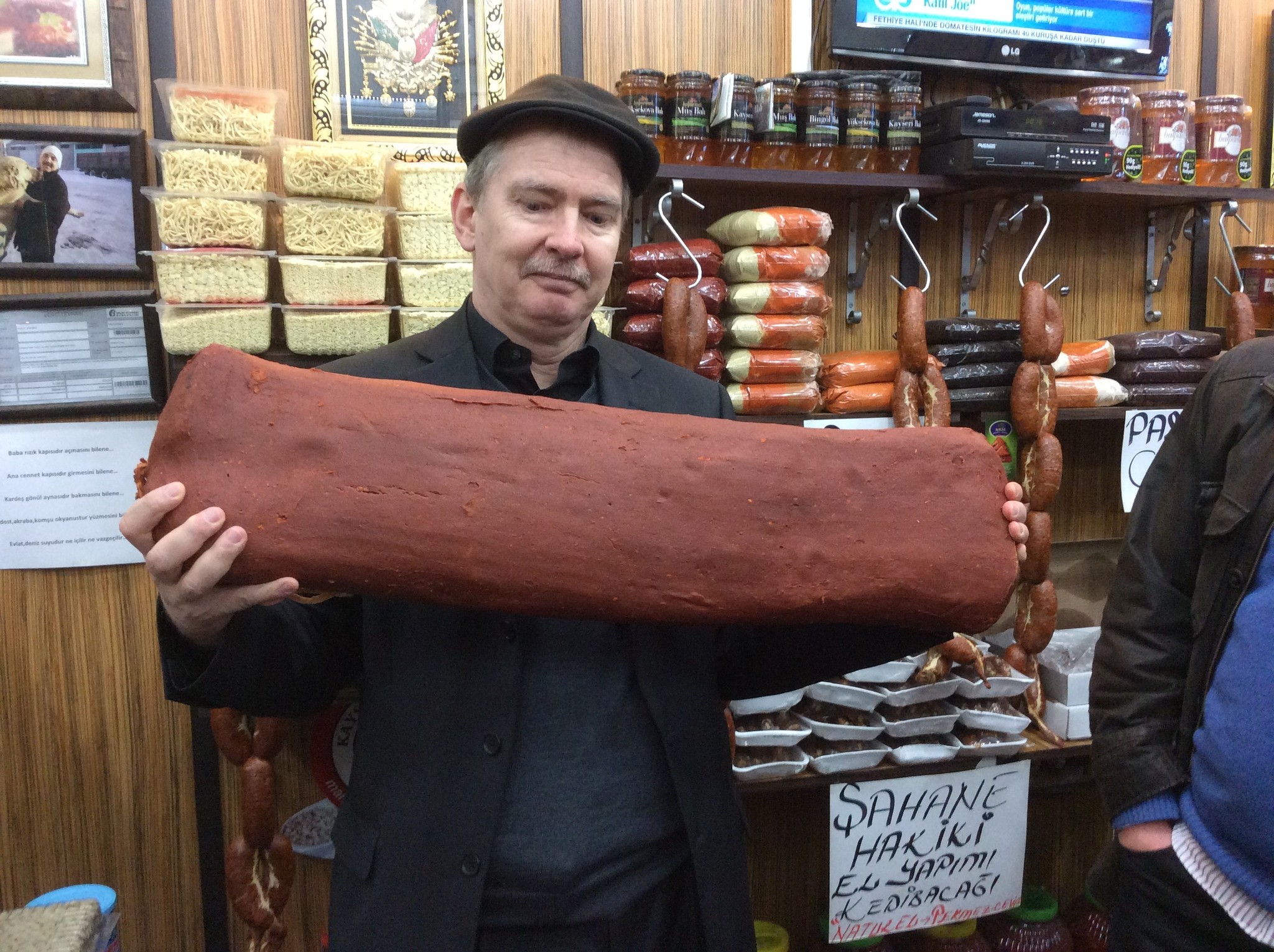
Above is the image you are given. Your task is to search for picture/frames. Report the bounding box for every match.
[0,290,168,418]
[304,0,506,163]
[0,122,154,280]
[0,0,140,113]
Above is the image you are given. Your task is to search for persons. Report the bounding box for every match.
[14,144,72,262]
[1087,332,1274,952]
[117,74,1031,952]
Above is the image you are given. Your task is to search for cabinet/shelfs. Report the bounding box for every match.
[658,165,952,430]
[962,179,1272,423]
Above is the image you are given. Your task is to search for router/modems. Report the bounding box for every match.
[914,138,1115,177]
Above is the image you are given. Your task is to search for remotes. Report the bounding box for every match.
[913,95,994,111]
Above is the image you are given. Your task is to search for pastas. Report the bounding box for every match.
[136,74,479,363]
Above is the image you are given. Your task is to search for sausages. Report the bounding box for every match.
[1228,288,1259,351]
[663,279,711,372]
[889,279,1064,745]
[205,704,299,950]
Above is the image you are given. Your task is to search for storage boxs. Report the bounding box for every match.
[985,625,1101,740]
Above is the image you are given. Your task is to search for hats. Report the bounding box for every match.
[456,74,662,198]
[39,145,64,169]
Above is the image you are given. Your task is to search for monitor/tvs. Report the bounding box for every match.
[830,0,1172,82]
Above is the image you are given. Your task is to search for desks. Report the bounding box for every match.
[742,722,1112,952]
